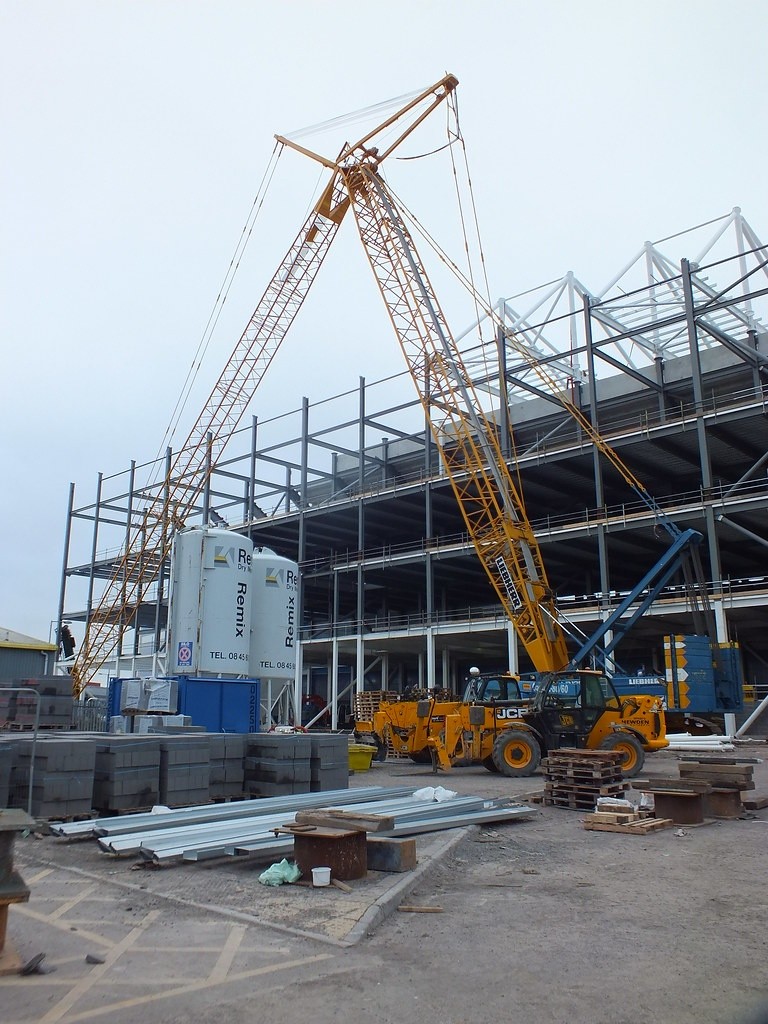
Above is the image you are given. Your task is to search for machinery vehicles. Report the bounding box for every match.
[64,71,748,786]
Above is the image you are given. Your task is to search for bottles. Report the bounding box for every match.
[280,728,302,734]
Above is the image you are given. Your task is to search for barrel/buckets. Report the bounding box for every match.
[311,867,331,886]
